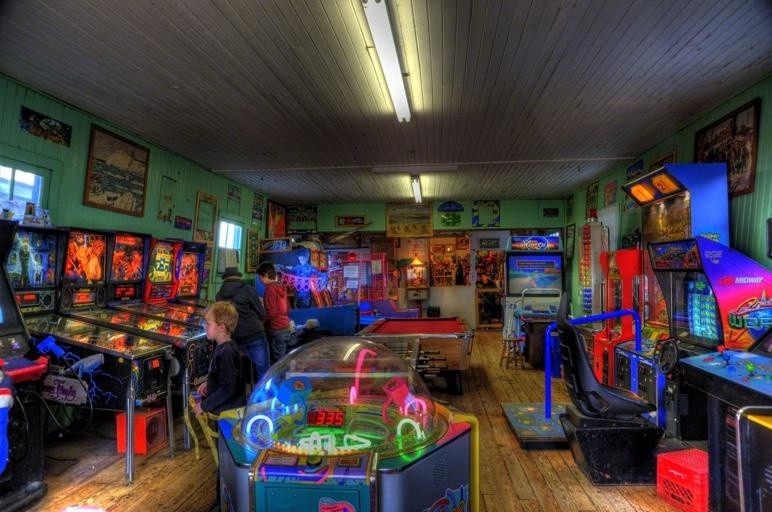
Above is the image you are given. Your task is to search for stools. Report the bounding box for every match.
[500,337,526,370]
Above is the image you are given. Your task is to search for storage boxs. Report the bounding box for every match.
[657,449,710,512]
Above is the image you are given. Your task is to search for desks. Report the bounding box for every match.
[354,317,474,396]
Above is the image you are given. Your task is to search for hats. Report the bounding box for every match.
[222,267,242,279]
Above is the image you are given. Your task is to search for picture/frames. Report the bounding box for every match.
[247,230,259,273]
[649,150,677,172]
[694,96,760,198]
[83,124,151,217]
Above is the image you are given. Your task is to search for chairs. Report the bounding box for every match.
[558,292,664,485]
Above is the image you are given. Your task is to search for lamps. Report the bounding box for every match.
[411,176,423,205]
[361,1,412,124]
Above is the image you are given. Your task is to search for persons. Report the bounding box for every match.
[452,257,502,324]
[257,262,291,363]
[216,267,270,383]
[193,299,248,512]
